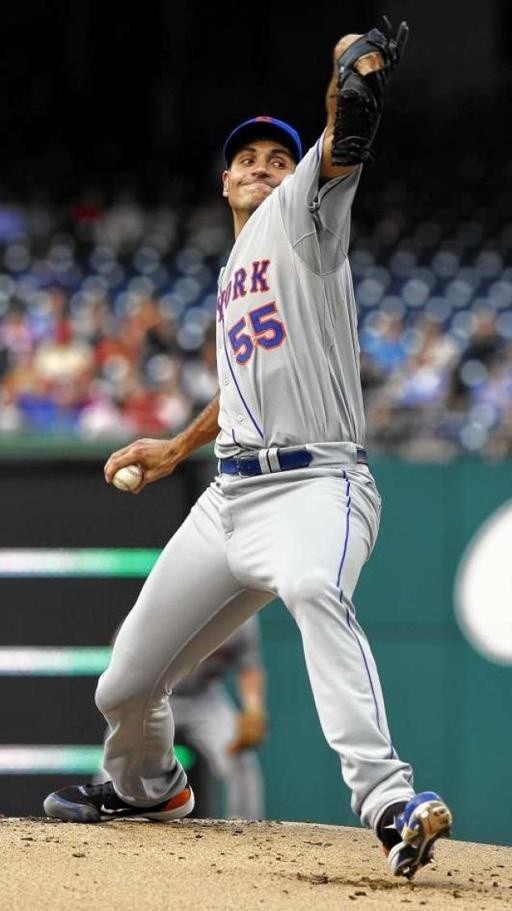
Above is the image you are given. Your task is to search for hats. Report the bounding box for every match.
[221,115,303,170]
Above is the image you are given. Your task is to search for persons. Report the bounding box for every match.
[42,32,453,879]
[168,611,268,821]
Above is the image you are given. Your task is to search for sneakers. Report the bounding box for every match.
[379,790,453,876]
[43,780,195,822]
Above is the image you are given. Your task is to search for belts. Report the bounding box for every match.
[220,447,369,478]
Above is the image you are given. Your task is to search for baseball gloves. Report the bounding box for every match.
[331,17,409,165]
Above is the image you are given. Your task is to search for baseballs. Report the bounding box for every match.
[113,464,142,490]
